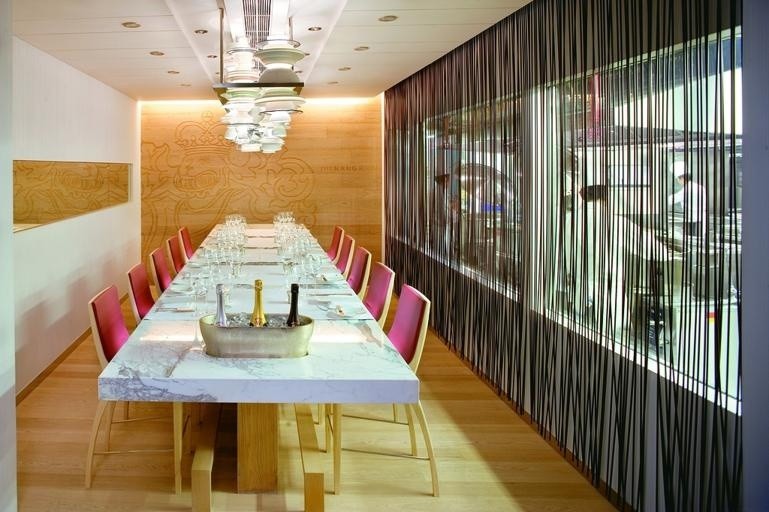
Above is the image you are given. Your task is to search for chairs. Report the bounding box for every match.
[319,262,396,422]
[85,284,190,496]
[326,227,373,303]
[150,227,197,296]
[128,263,206,423]
[326,284,439,496]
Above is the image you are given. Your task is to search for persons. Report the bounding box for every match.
[429,173,459,260]
[563,186,668,331]
[669,161,708,236]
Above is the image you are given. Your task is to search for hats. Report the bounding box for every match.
[671,161,692,177]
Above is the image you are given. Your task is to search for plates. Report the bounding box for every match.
[170,284,192,294]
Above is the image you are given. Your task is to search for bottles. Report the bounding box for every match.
[286,283,300,326]
[249,279,269,328]
[214,282,227,326]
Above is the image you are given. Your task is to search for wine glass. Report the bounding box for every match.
[271,210,323,308]
[189,212,249,318]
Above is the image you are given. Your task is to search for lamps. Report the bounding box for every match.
[216,1,305,154]
